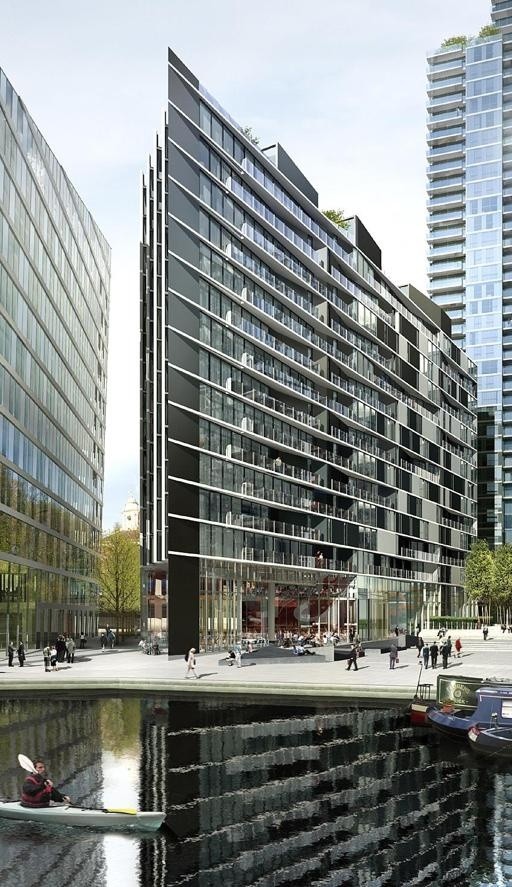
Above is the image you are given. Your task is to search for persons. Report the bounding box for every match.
[185,648,199,680]
[228,624,512,672]
[143,640,149,654]
[22,758,71,808]
[137,640,142,650]
[152,639,160,656]
[4,627,120,673]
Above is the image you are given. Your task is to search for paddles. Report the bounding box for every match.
[70,803,136,814]
[19,752,75,804]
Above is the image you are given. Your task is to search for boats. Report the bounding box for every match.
[1,815,159,840]
[410,664,512,727]
[428,720,469,751]
[468,712,512,758]
[0,796,167,832]
[426,673,512,736]
[460,742,512,772]
